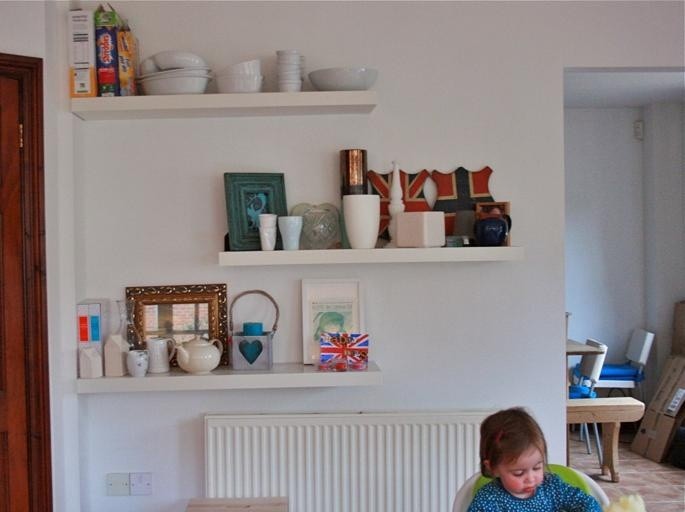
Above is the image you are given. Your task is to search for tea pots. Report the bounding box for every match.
[173,334,222,374]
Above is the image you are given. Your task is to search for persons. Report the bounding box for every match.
[466,407,603,512]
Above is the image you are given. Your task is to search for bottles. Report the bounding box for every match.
[115,298,143,351]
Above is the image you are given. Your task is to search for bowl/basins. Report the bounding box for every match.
[308,67,377,91]
[136,50,211,93]
[275,50,303,91]
[216,60,263,92]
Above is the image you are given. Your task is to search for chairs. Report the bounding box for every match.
[569,339,608,469]
[454,464,608,511]
[574,327,656,403]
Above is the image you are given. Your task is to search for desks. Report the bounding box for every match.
[567,339,603,356]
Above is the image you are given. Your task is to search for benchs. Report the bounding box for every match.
[566,396,645,482]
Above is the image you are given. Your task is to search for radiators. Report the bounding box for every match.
[202,410,493,511]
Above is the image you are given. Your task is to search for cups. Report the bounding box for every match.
[257,214,278,251]
[276,216,303,250]
[343,194,381,249]
[147,337,175,373]
[126,350,149,379]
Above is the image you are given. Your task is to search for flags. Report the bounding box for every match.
[320,333,368,368]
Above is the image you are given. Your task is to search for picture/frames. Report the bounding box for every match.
[124,284,229,368]
[223,171,289,251]
